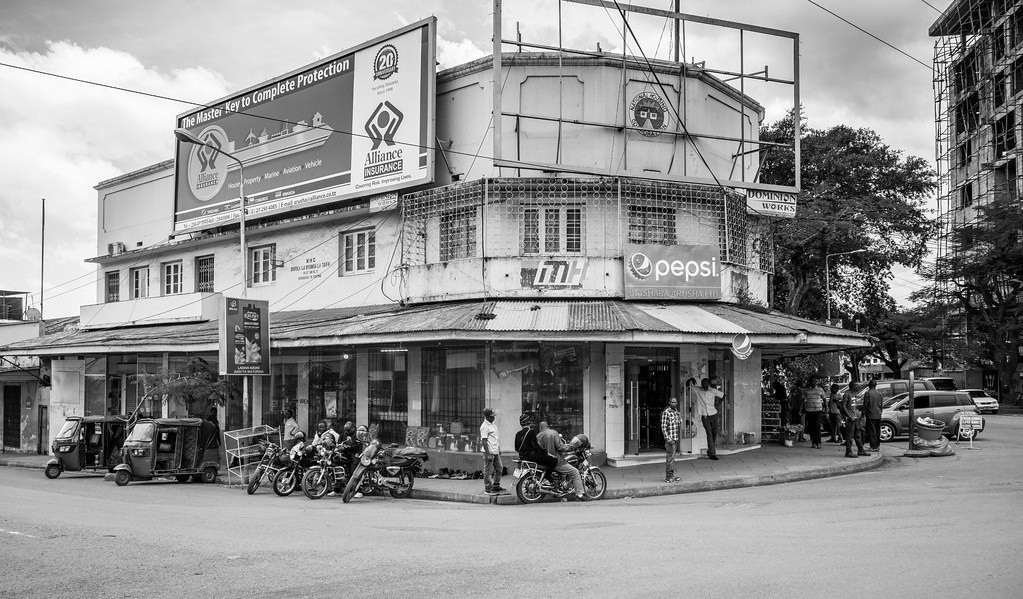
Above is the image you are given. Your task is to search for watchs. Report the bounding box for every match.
[826,406,829,409]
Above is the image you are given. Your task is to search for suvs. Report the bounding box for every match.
[876,390,986,443]
[919,377,957,391]
[958,388,1000,415]
[831,381,936,410]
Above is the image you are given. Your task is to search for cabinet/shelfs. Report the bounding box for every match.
[223,424,284,489]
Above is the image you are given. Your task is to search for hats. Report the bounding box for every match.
[483,407,497,417]
[520,413,530,426]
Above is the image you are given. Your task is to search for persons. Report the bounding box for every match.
[310,421,338,455]
[825,384,846,442]
[839,379,871,458]
[208,407,221,448]
[859,376,883,453]
[685,377,725,460]
[661,396,683,483]
[327,421,365,498]
[282,410,300,452]
[514,414,559,489]
[804,375,828,450]
[535,421,593,503]
[772,379,803,428]
[479,408,512,496]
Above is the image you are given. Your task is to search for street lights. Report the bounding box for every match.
[178,130,251,427]
[826,248,868,318]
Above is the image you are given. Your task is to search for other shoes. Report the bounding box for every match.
[826,437,836,442]
[811,444,817,448]
[870,447,879,452]
[845,451,858,458]
[709,455,719,461]
[817,444,822,449]
[798,436,807,442]
[858,450,871,456]
[836,437,843,443]
[706,450,710,455]
[841,441,852,446]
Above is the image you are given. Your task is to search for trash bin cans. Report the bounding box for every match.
[915,418,946,441]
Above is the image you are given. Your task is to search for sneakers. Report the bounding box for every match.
[665,476,680,483]
[542,479,552,485]
[485,489,498,495]
[576,495,591,502]
[493,486,506,493]
[353,492,364,499]
[327,491,339,497]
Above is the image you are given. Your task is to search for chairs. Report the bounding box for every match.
[157,438,174,465]
[87,435,101,454]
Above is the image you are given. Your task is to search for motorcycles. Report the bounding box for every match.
[272,442,367,499]
[511,434,607,503]
[44,415,131,479]
[342,441,430,505]
[249,441,307,497]
[112,418,222,485]
